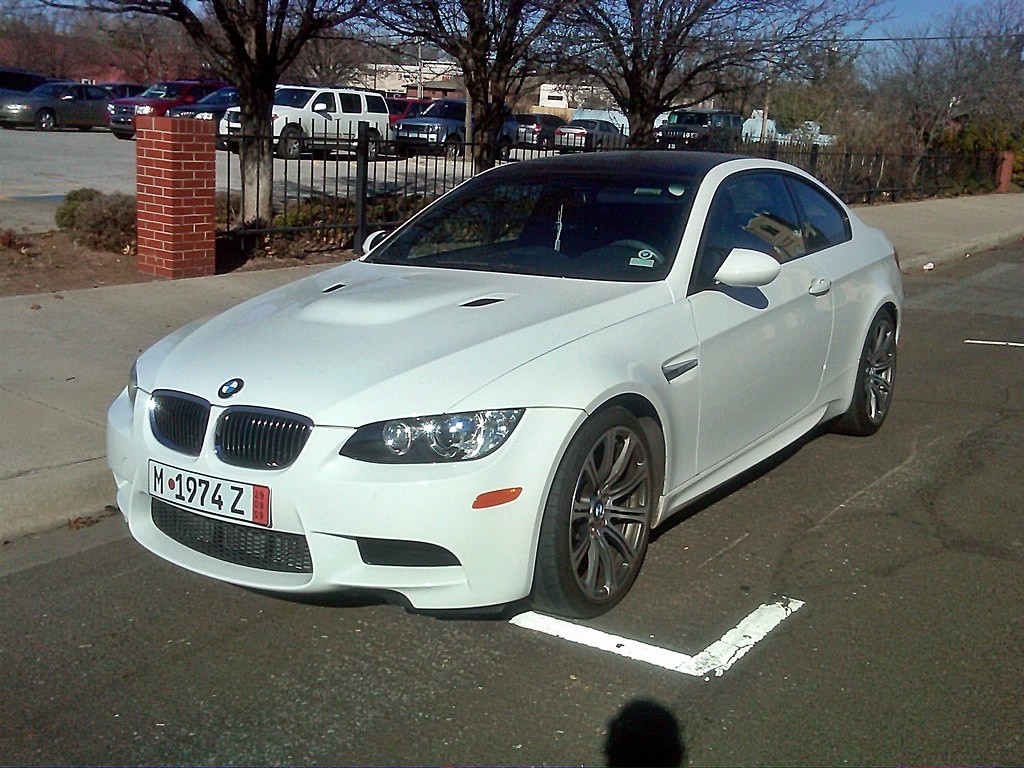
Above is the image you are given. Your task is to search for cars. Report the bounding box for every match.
[163,84,240,151]
[0,81,117,133]
[104,152,904,621]
[554,118,630,153]
[92,81,150,102]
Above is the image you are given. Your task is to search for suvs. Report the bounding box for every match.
[219,84,390,162]
[511,112,567,150]
[653,107,745,152]
[390,97,519,164]
[0,67,52,113]
[382,96,435,123]
[106,77,236,142]
